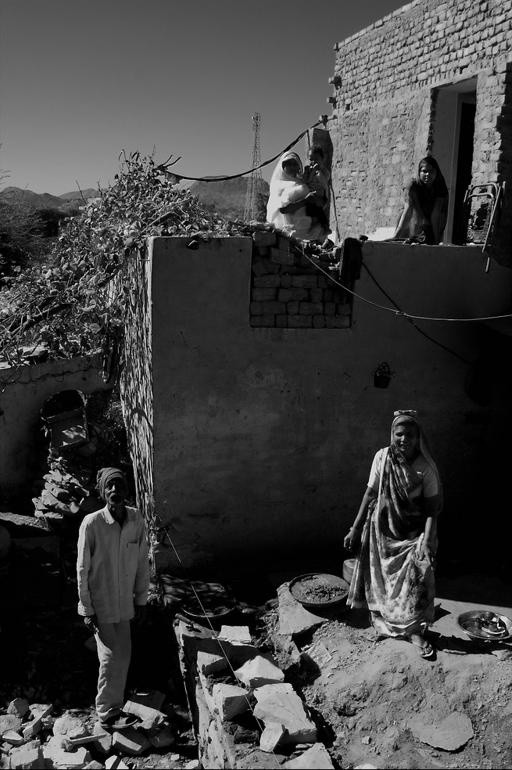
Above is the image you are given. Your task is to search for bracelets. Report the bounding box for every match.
[349,527,361,533]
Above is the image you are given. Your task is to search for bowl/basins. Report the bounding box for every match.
[456,609,512,645]
[289,573,350,607]
[180,592,238,622]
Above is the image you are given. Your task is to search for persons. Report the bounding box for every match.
[265,151,331,245]
[302,144,333,237]
[385,156,449,245]
[343,408,445,659]
[74,468,152,731]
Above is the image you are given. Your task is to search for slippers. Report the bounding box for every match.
[101,713,139,730]
[411,637,434,659]
